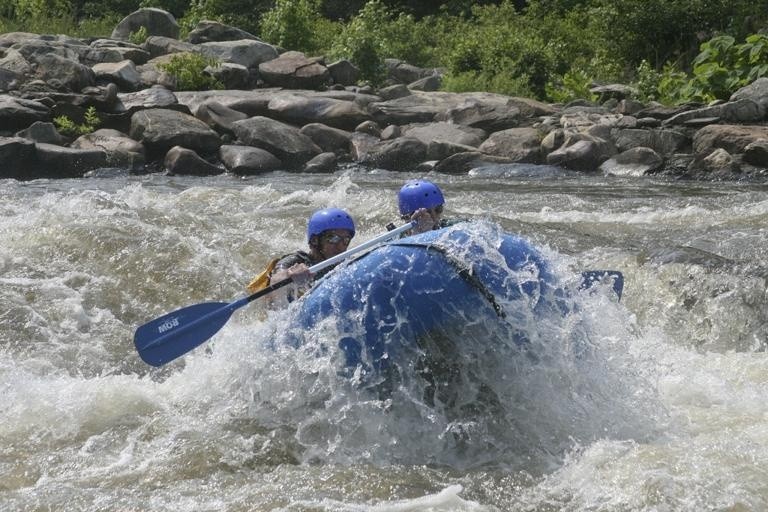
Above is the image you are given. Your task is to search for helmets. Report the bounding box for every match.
[307,208,355,244]
[398,179,445,214]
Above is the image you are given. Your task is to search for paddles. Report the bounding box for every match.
[580,270,623,300]
[133,221,416,366]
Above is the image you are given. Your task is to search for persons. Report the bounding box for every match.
[267,207,356,310]
[396,177,472,239]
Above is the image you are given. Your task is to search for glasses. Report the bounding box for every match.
[324,233,351,246]
[427,205,443,214]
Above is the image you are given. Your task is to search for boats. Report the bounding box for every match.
[220,224,591,400]
